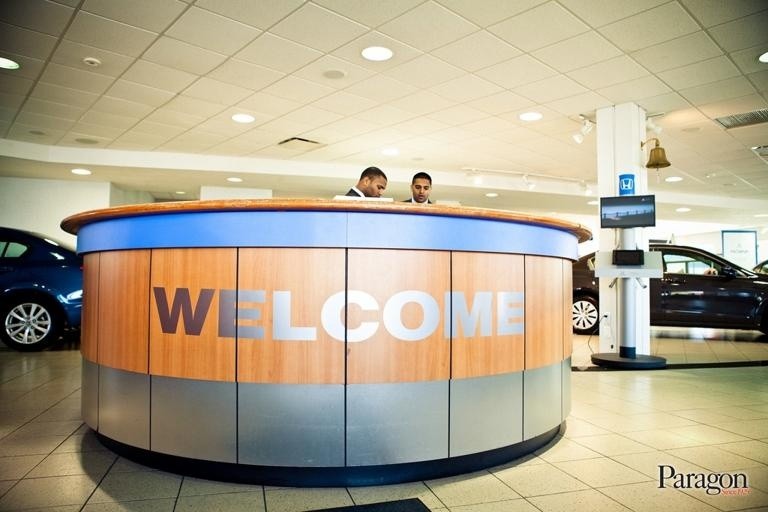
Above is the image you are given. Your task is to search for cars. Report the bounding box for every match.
[0,226,82,352]
[573,244,768,335]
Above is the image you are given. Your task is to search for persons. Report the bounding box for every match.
[401,171,433,204]
[347,166,388,197]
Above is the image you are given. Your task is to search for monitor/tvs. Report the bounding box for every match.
[600,194,655,228]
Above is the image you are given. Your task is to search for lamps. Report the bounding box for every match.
[573,119,593,144]
[640,138,672,172]
